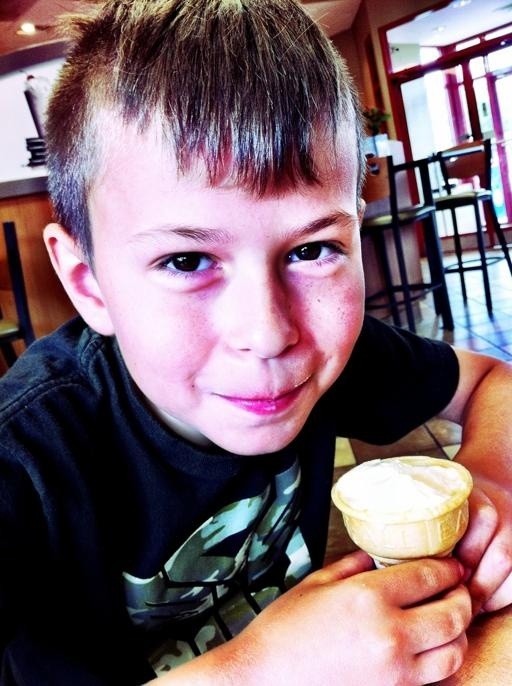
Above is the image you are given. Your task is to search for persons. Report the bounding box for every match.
[0,0,511,686]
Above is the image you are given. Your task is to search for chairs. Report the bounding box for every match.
[360,137,507,337]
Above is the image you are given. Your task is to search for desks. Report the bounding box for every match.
[324,416,512,686]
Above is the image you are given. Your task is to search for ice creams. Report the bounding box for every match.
[330,454,475,570]
